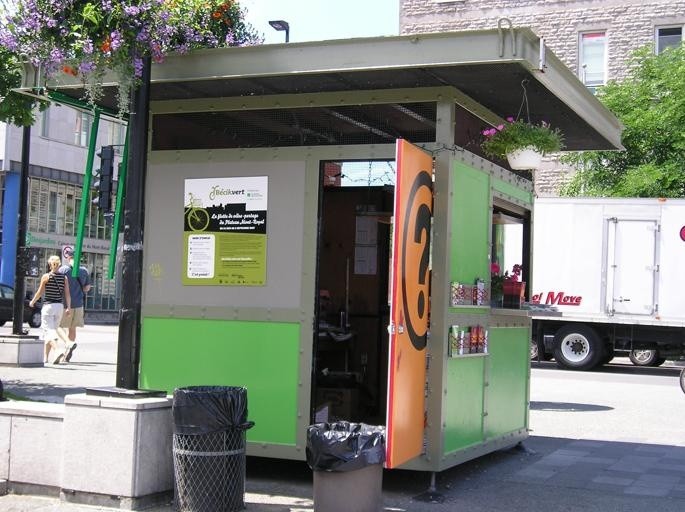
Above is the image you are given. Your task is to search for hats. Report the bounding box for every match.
[68,250,75,256]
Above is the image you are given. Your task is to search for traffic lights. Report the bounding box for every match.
[92,146,114,213]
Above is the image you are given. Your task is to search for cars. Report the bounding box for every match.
[0,283,42,329]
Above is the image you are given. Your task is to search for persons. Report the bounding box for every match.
[56,251,92,364]
[29,254,71,365]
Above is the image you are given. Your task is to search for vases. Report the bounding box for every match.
[505,144,544,170]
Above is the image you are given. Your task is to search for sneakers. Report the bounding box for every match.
[44,341,77,364]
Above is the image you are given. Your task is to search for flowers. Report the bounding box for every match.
[479,117,567,162]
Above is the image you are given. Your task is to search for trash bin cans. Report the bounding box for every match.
[308,420,386,511]
[171,386,246,511]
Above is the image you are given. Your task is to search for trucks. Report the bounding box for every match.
[504,196,685,372]
